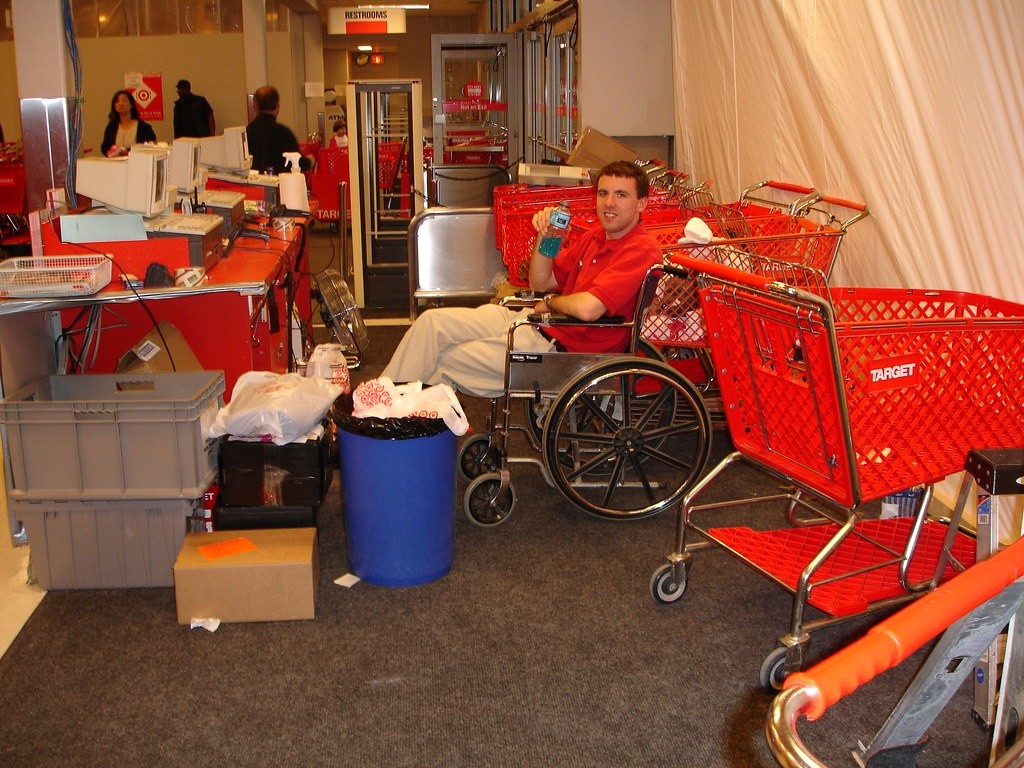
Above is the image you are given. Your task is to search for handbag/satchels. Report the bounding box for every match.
[305,343,350,395]
[354,379,469,437]
[210,370,344,447]
[640,218,751,343]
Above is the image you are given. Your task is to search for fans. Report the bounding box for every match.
[316,270,370,370]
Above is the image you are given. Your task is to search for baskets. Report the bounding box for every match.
[0,254,114,300]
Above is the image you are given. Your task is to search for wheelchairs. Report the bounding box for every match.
[454,262,715,532]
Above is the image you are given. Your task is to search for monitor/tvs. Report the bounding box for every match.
[131,136,204,202]
[198,126,259,183]
[75,146,178,233]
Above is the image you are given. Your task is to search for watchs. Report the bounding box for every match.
[543,293,558,311]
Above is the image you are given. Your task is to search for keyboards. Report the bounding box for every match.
[204,189,246,209]
[160,213,224,235]
[248,175,280,187]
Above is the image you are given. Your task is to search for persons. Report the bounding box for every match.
[245,85,316,175]
[172,79,215,139]
[378,161,665,400]
[327,118,348,151]
[100,88,157,157]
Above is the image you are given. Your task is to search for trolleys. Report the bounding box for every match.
[490,155,870,434]
[646,251,1023,697]
[296,129,508,235]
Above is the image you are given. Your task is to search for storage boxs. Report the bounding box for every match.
[214,418,335,543]
[567,127,638,168]
[173,527,320,624]
[115,318,204,391]
[0,370,225,500]
[13,500,206,591]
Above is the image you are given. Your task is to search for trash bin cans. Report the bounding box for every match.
[333,383,457,586]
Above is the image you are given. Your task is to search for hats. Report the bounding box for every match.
[176,80,191,89]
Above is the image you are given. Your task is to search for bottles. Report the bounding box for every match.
[538,201,571,259]
